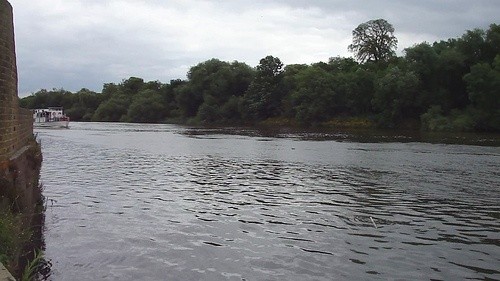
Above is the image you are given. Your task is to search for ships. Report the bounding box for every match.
[32,106,70,128]
